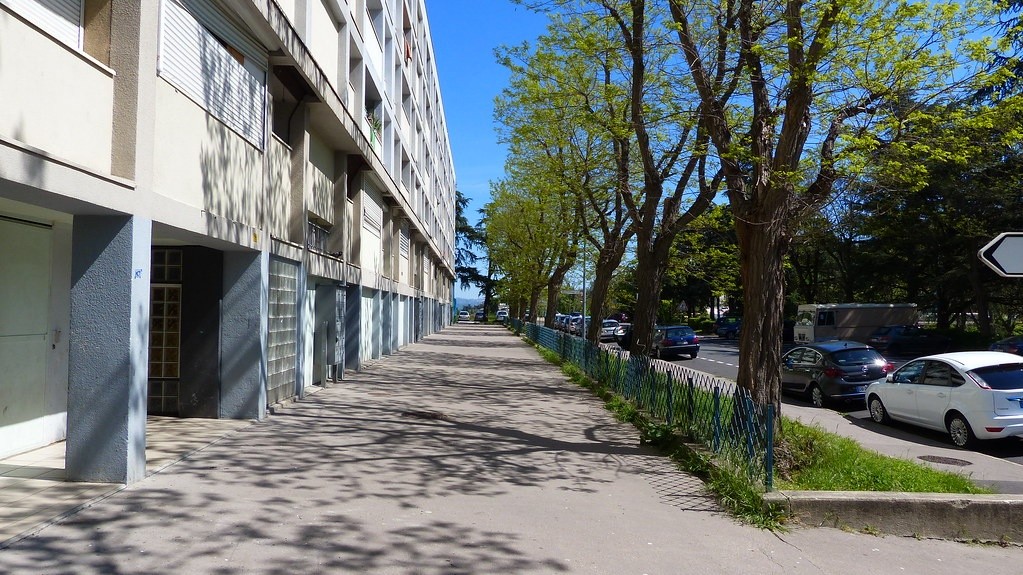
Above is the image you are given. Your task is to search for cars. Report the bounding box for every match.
[651,325,700,360]
[575,316,591,337]
[475,313,485,321]
[525,310,530,321]
[867,324,936,354]
[782,341,894,408]
[614,323,634,350]
[554,314,582,334]
[458,311,470,321]
[713,317,742,340]
[496,311,508,322]
[864,351,1023,448]
[988,334,1023,356]
[601,319,620,342]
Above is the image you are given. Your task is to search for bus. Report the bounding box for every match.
[793,302,917,344]
